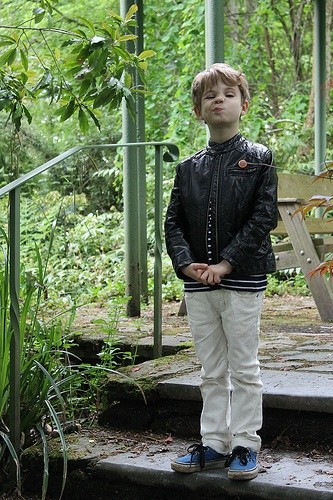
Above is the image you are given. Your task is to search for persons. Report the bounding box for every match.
[164,62,281,480]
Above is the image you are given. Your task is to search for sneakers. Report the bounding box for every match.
[171,443,259,480]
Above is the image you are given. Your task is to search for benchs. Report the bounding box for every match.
[176,173,333,326]
